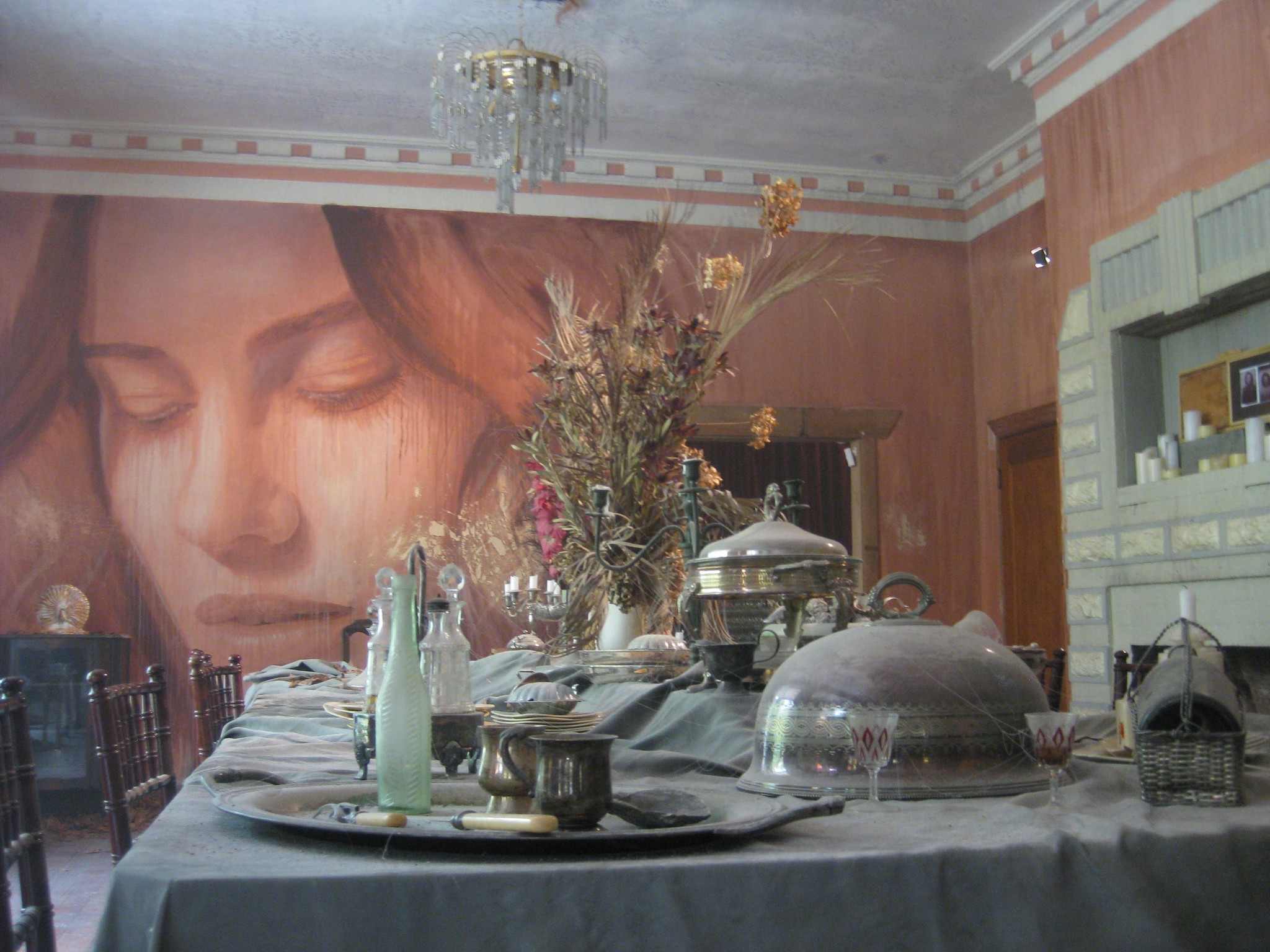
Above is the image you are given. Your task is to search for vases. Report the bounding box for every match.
[594,583,647,649]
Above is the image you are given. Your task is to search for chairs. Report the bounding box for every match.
[187,647,244,766]
[0,675,63,952]
[84,660,174,865]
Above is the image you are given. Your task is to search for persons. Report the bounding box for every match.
[1241,372,1270,405]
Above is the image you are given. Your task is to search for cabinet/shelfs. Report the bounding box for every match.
[0,630,134,819]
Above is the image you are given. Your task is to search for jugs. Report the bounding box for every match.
[689,628,780,682]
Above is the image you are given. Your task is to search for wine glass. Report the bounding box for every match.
[1024,711,1079,812]
[846,710,902,814]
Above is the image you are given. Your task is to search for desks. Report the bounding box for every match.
[89,662,1270,952]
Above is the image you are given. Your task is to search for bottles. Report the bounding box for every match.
[362,561,471,817]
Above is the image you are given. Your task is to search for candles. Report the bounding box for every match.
[510,574,519,590]
[529,573,539,589]
[553,583,561,595]
[546,578,555,592]
[503,584,510,595]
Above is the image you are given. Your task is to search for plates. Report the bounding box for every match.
[573,649,695,686]
[322,700,603,735]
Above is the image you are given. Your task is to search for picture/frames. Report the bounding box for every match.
[1226,344,1270,427]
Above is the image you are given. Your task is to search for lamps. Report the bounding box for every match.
[424,0,609,219]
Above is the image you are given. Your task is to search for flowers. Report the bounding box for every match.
[502,157,887,641]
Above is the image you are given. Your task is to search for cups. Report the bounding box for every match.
[474,724,621,828]
